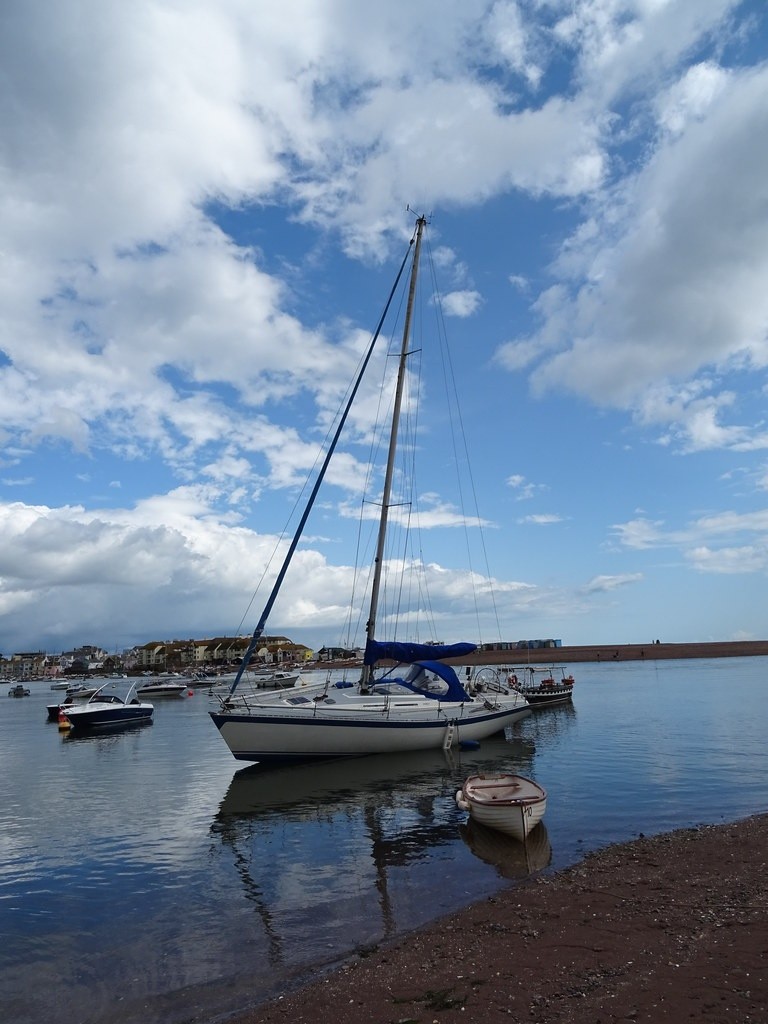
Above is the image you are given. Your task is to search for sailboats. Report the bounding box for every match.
[204,212,536,759]
[213,736,537,969]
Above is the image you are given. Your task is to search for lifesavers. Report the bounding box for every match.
[508,673,518,688]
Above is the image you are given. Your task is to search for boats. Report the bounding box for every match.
[8,684,31,696]
[45,697,79,717]
[51,682,70,690]
[65,681,98,698]
[459,812,556,884]
[42,676,67,682]
[186,671,216,688]
[495,665,574,708]
[142,671,153,677]
[456,769,548,838]
[158,672,174,678]
[180,666,218,676]
[136,679,188,697]
[216,664,232,675]
[63,680,155,726]
[110,675,124,680]
[297,666,312,673]
[252,669,300,689]
